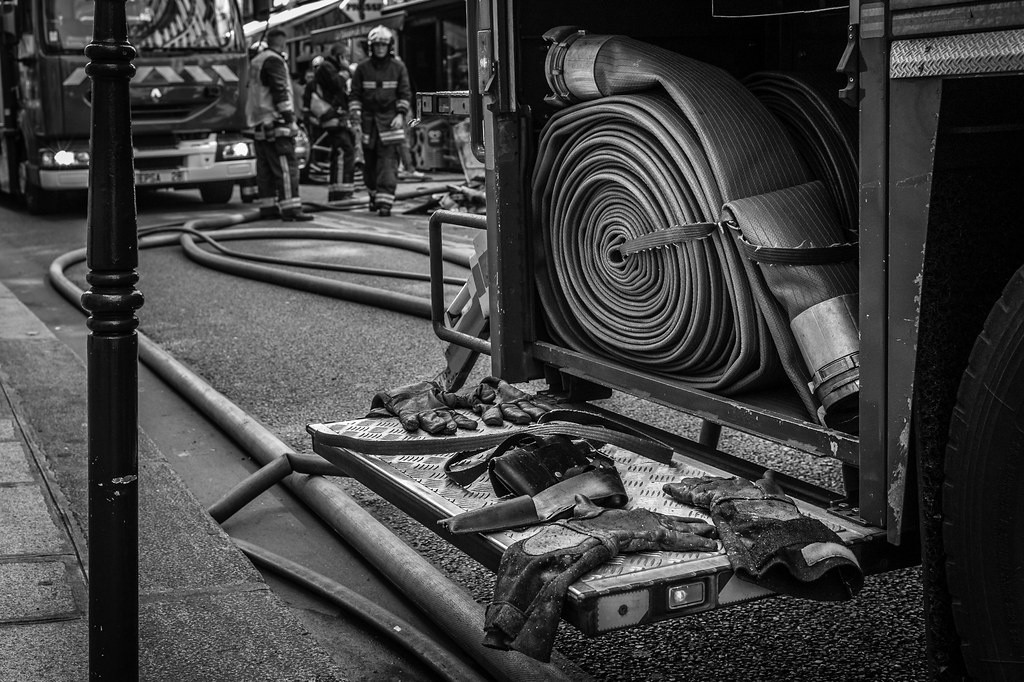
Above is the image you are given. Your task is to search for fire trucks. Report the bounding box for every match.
[306,0,1024,681]
[0,0,310,216]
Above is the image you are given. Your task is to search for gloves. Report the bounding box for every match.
[483,492,720,661]
[475,376,552,427]
[663,469,862,601]
[366,381,474,435]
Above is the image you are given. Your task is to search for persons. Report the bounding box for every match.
[290,40,367,202]
[244,30,314,222]
[349,25,411,217]
[397,110,423,177]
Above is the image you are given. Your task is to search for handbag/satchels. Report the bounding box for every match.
[438,431,626,536]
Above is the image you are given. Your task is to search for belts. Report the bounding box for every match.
[314,408,673,466]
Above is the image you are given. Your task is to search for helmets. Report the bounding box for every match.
[367,24,394,46]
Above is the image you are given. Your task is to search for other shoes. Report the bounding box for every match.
[281,208,313,221]
[369,201,376,211]
[379,205,391,216]
[261,206,281,220]
